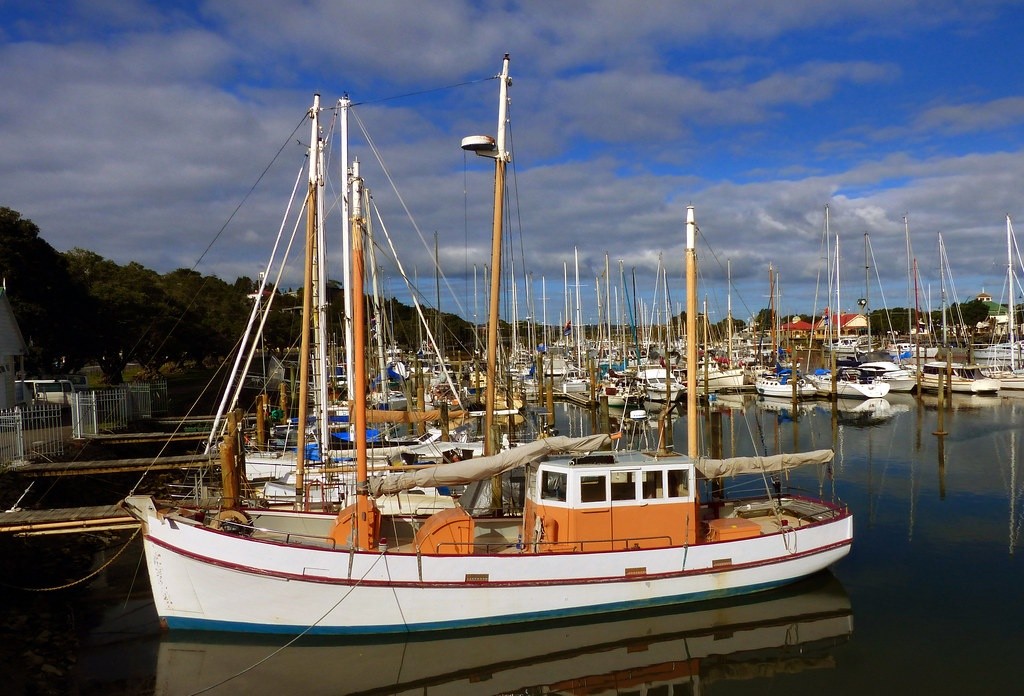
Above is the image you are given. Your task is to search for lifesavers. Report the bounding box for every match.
[210,511,250,534]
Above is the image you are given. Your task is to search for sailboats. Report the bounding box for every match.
[921,230,1002,396]
[150,575,861,696]
[126,52,855,639]
[204,93,969,511]
[972,213,1024,395]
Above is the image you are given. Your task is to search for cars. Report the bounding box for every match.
[14,379,75,404]
